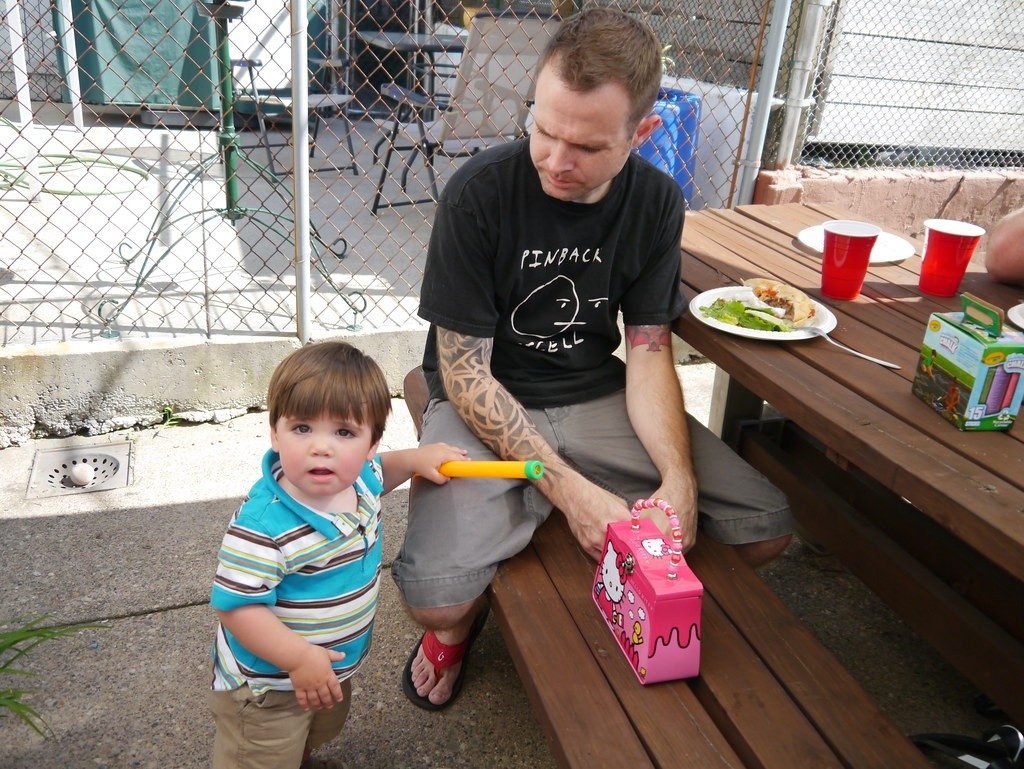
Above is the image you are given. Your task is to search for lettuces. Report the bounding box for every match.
[698,299,790,332]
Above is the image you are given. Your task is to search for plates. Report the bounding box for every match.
[1007,303,1024,329]
[688,285,837,340]
[798,224,916,263]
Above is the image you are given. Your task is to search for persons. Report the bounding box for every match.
[386,5,797,714]
[982,200,1024,290]
[206,341,472,769]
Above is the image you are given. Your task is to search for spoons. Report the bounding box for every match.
[795,327,902,370]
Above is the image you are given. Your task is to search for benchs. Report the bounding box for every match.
[403,363,937,769]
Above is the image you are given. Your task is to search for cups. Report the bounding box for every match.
[918,219,986,297]
[822,219,881,300]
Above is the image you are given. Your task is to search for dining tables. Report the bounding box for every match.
[355,30,498,164]
[633,193,1024,718]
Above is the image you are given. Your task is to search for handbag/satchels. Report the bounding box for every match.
[594,498,703,686]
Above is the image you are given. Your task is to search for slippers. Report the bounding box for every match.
[401,591,491,712]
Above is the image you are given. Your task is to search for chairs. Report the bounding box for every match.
[230,57,308,180]
[367,80,450,216]
[305,58,362,178]
